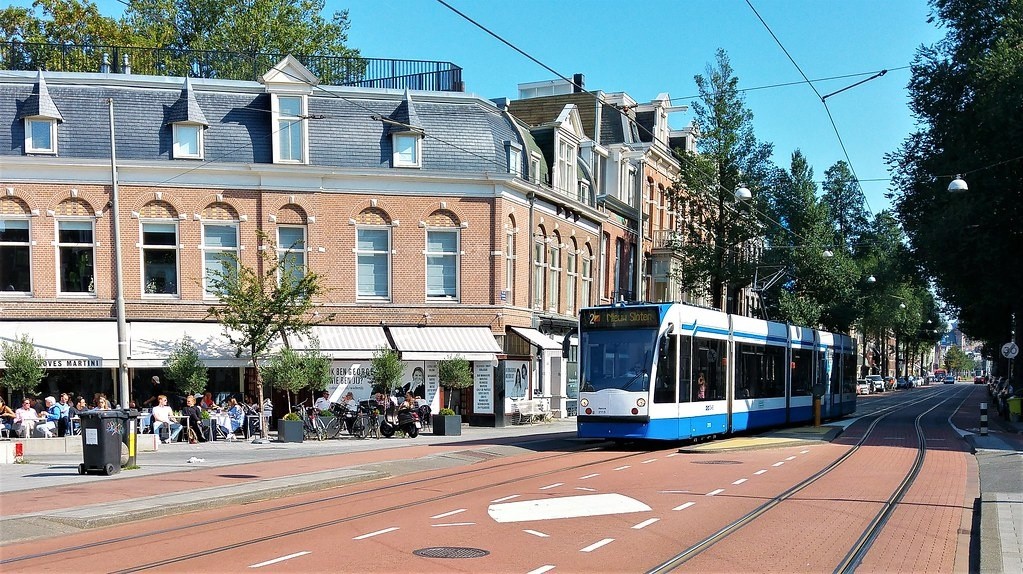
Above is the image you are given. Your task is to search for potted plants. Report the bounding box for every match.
[276,411,305,444]
[199,410,218,442]
[361,407,385,435]
[317,410,340,438]
[432,406,463,437]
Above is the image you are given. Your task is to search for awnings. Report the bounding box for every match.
[0,319,563,362]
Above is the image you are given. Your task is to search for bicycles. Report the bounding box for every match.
[291,396,434,442]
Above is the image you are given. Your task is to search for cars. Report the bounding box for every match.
[943,376,955,384]
[974,376,985,384]
[856,368,955,395]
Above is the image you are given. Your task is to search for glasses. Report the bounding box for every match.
[26,403,30,404]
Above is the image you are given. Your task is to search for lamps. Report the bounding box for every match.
[821,248,834,260]
[899,302,906,309]
[926,319,932,324]
[946,173,970,194]
[734,181,754,201]
[867,274,876,284]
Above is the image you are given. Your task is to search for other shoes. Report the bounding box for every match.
[226,434,231,439]
[164,439,170,443]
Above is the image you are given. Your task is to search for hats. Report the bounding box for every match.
[321,389,328,392]
[347,392,352,395]
[152,376,160,384]
[193,393,204,398]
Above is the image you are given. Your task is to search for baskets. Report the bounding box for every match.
[328,402,348,417]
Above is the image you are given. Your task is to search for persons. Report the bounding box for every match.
[697,373,705,399]
[628,361,648,377]
[402,366,425,398]
[511,363,529,396]
[0,392,428,444]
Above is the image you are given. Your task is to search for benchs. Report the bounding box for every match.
[515,400,552,426]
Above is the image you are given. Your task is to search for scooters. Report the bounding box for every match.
[380,397,422,439]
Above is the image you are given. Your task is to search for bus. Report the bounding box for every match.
[561,261,859,447]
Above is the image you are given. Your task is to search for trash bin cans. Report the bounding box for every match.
[1007,396,1023,416]
[77,410,126,474]
